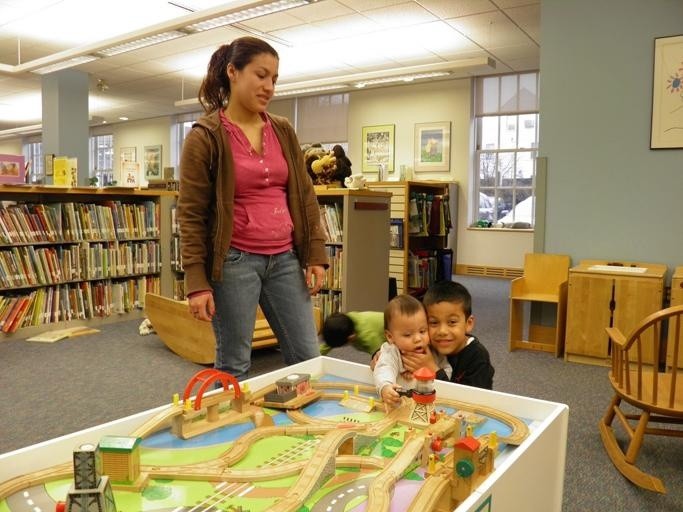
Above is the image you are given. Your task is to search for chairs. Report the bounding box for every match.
[508,253,571,358]
[599,304,683,493]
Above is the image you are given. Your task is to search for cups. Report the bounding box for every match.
[378,163,388,182]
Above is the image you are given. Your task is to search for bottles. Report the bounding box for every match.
[399,164,413,181]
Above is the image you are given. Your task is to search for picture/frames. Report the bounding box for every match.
[45,154,55,175]
[143,145,162,179]
[414,121,451,172]
[120,147,136,162]
[361,124,395,172]
[121,162,140,185]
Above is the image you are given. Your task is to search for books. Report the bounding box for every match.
[0,198,160,344]
[405,188,454,297]
[303,197,346,333]
[169,206,188,300]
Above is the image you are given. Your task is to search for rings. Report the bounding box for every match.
[192,311,199,314]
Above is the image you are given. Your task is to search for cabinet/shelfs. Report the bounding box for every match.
[1,186,164,341]
[159,191,392,317]
[664,266,682,373]
[563,259,668,375]
[416,180,459,274]
[365,182,446,304]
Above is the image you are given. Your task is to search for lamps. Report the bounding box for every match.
[0,125,42,140]
[174,59,496,111]
[30,1,321,75]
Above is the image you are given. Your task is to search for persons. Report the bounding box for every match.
[319,309,387,357]
[372,294,453,416]
[175,36,331,389]
[370,279,495,389]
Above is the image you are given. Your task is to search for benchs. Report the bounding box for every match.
[144,291,321,366]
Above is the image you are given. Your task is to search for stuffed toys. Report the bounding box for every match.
[302,142,367,188]
